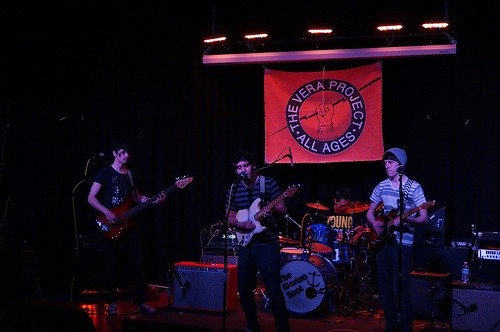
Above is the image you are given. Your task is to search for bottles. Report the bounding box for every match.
[462,262,469,284]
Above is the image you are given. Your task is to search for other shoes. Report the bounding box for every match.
[139,304,156,316]
[107,302,118,315]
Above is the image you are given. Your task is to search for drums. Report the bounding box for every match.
[304,223,340,254]
[277,253,342,315]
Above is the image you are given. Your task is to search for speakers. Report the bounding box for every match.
[172,261,239,314]
[450,278,500,332]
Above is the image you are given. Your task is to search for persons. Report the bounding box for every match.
[366,148,429,332]
[226,152,291,332]
[321,188,370,245]
[88,139,168,316]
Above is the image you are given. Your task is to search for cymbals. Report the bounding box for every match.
[277,234,303,246]
[334,201,369,214]
[305,203,331,210]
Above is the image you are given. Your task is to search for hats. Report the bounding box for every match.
[385,147,407,168]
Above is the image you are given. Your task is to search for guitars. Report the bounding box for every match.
[369,198,435,247]
[95,174,196,240]
[234,182,301,248]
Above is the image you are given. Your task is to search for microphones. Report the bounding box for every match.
[454,301,478,318]
[288,146,293,167]
[396,165,402,173]
[179,281,192,302]
[93,152,105,158]
